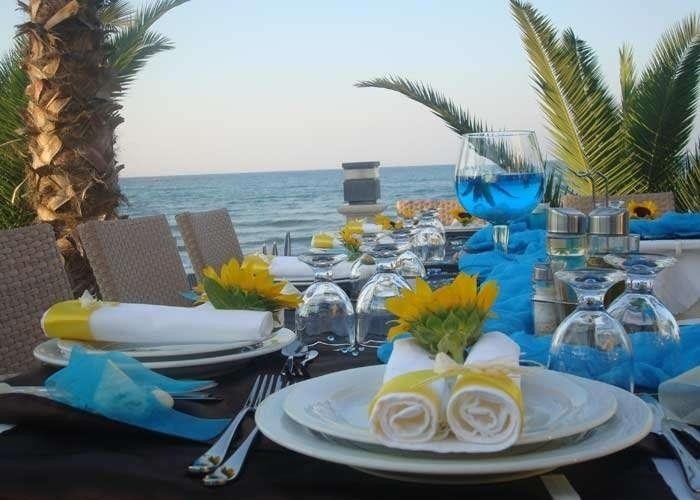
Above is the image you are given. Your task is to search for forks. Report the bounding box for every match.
[190,372,290,485]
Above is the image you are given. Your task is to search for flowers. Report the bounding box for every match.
[337,203,480,257]
[382,269,502,359]
[200,257,304,325]
[624,199,660,221]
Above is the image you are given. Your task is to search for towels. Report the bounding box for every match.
[36,292,273,346]
[374,327,530,453]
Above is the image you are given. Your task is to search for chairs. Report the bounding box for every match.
[78,214,195,320]
[173,206,245,283]
[0,222,77,384]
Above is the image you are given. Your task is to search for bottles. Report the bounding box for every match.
[534,267,559,336]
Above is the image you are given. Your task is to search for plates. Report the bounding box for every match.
[283,364,618,455]
[253,364,657,483]
[28,329,291,369]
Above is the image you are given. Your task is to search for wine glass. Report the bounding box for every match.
[547,252,680,396]
[295,205,444,351]
[452,129,548,253]
